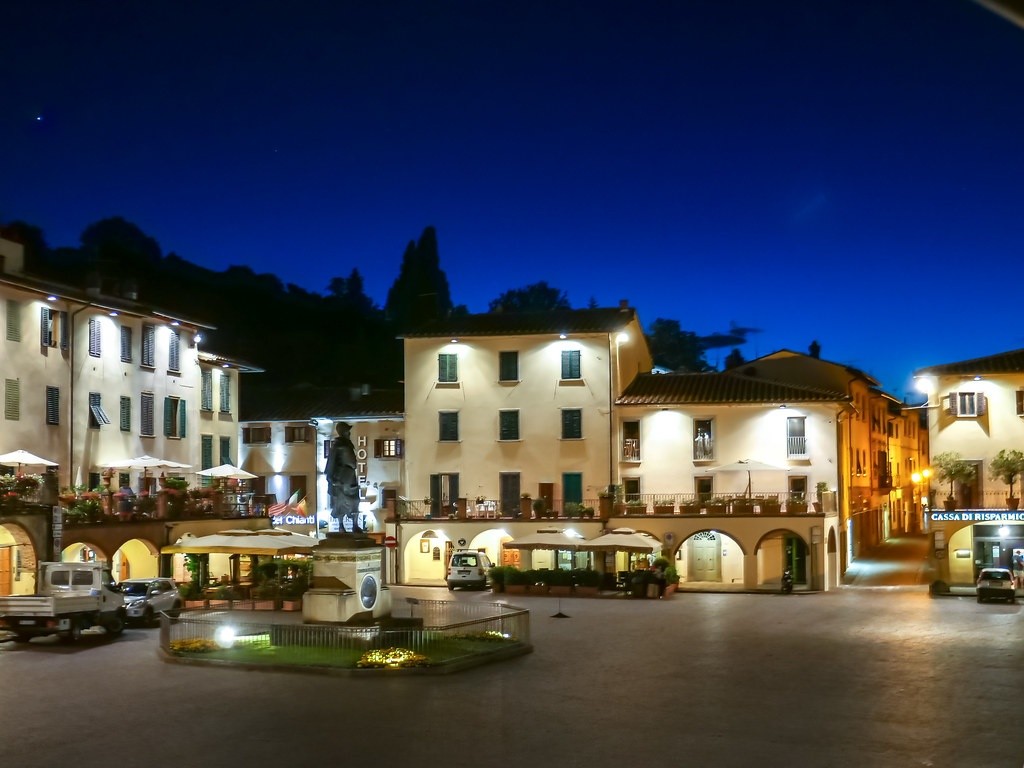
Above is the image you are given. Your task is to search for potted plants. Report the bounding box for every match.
[598,489,610,519]
[487,566,602,598]
[932,452,974,511]
[757,499,784,514]
[785,497,808,514]
[626,500,647,515]
[705,497,726,514]
[612,483,625,515]
[520,492,532,519]
[61,478,224,522]
[647,559,680,597]
[812,482,837,518]
[678,500,702,514]
[183,557,313,611]
[987,450,1024,512]
[652,499,674,514]
[533,499,545,519]
[731,499,754,514]
[565,502,594,519]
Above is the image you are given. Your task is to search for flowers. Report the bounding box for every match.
[0,473,44,505]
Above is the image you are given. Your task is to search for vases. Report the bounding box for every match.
[547,510,558,520]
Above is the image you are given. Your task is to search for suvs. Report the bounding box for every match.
[119,578,184,628]
[975,568,1018,603]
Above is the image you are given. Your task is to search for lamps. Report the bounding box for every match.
[422,530,438,539]
[780,404,786,409]
[309,420,318,427]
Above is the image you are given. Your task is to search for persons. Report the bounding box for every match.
[1010,550,1024,590]
[324,422,364,532]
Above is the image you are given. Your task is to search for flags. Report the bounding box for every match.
[267,490,301,517]
[291,496,311,518]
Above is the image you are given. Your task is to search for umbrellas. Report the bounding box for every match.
[195,463,260,492]
[0,449,60,480]
[96,454,193,497]
[580,527,663,576]
[159,529,296,594]
[254,529,321,586]
[703,458,795,499]
[502,525,589,571]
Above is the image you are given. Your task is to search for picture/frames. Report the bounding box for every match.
[420,539,430,553]
[676,550,681,560]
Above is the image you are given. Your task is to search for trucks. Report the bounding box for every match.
[0,561,128,644]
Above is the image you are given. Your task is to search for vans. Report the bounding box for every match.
[446,550,496,591]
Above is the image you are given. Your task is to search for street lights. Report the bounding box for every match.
[616,333,628,400]
